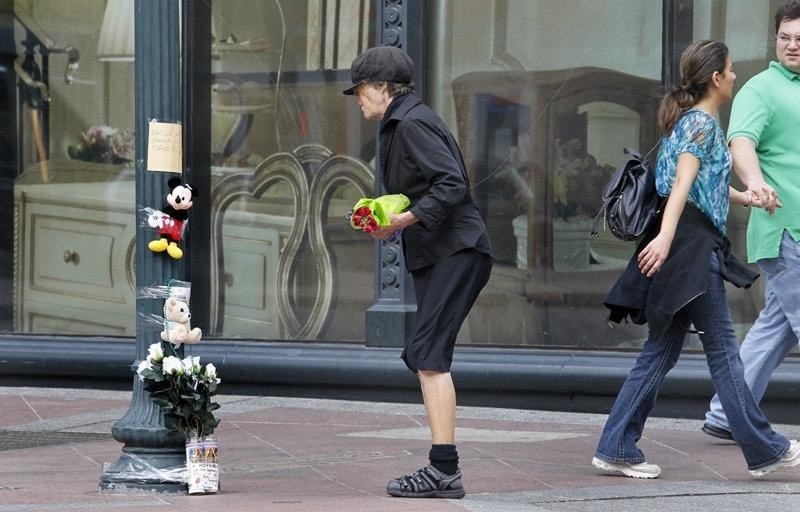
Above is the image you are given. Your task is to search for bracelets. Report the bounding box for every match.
[742,190,752,210]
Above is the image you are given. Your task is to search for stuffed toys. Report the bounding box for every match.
[160,297,203,346]
[148,175,199,263]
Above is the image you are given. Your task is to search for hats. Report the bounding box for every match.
[340,44,417,96]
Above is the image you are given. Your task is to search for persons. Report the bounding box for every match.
[700,0,800,440]
[341,46,495,502]
[591,37,800,480]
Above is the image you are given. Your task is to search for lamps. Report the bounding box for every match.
[96,0,221,61]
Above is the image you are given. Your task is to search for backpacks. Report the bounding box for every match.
[602,110,722,245]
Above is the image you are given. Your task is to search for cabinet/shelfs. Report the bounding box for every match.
[14,190,374,341]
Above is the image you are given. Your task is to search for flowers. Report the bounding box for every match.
[503,132,609,215]
[136,336,233,436]
[77,125,136,168]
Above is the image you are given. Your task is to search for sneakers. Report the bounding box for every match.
[701,419,736,449]
[589,450,662,480]
[386,463,466,500]
[744,438,800,479]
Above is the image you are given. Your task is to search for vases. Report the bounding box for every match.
[510,214,593,271]
[181,428,220,499]
